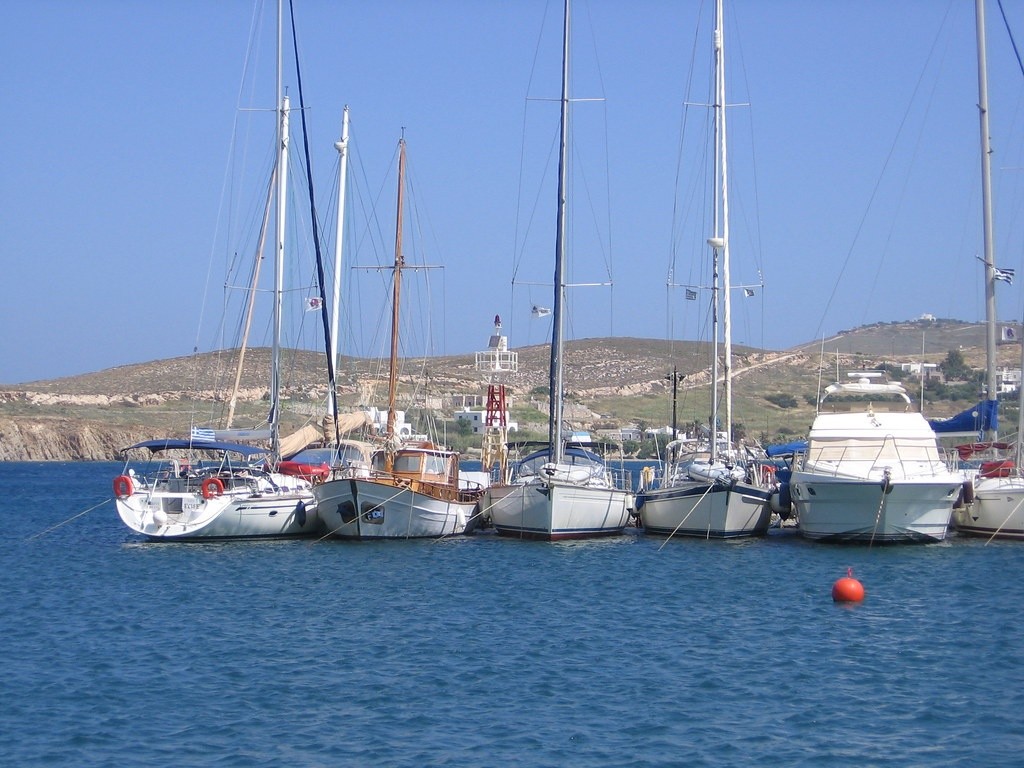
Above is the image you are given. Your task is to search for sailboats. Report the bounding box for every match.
[113,1,1023,540]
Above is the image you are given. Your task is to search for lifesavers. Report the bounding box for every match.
[202,478,223,498]
[759,465,772,479]
[113,476,133,497]
[643,467,651,484]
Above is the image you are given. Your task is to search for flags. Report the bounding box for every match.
[305,298,323,312]
[745,289,755,297]
[533,306,551,318]
[993,268,1015,286]
[685,288,697,300]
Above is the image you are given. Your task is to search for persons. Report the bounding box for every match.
[162,469,170,483]
[180,466,192,480]
